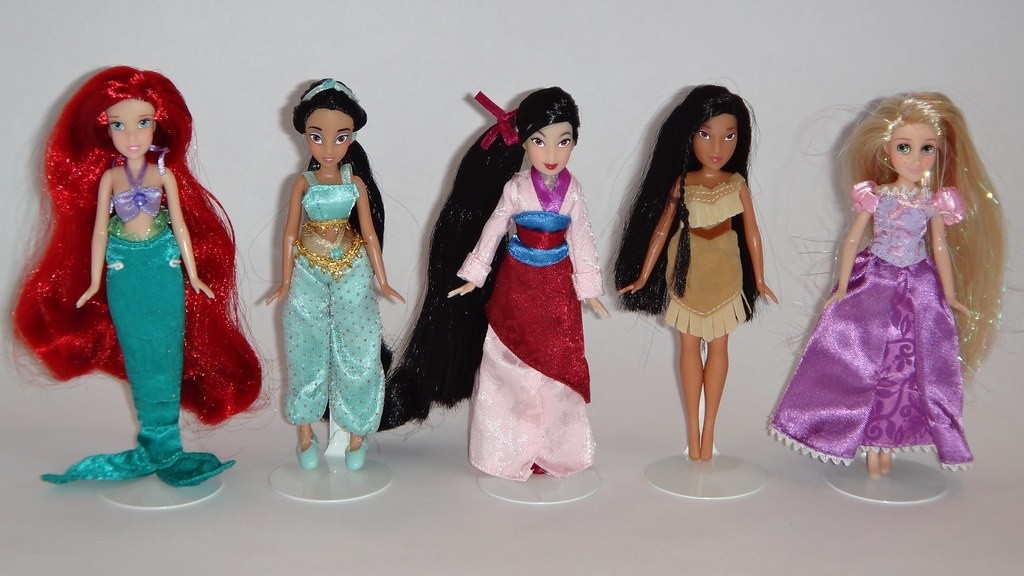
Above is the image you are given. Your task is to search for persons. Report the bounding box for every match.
[614,85,779,462]
[267,77,406,470]
[381,87,609,482]
[7,66,262,488]
[770,90,1006,477]
